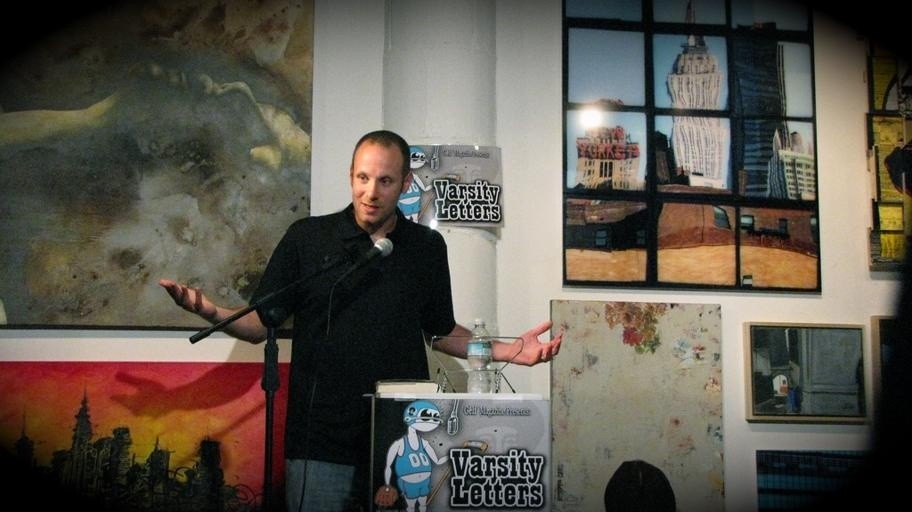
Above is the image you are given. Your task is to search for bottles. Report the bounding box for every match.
[466,318,492,396]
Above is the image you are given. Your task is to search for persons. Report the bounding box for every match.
[603,459,676,512]
[786,358,801,413]
[159,129,565,512]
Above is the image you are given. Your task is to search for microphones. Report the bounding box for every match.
[334,236,394,286]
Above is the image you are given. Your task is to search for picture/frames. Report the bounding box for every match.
[741,321,872,425]
[558,0,824,294]
